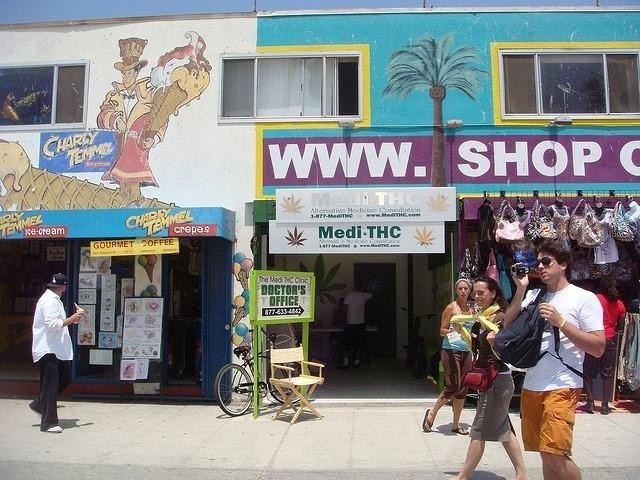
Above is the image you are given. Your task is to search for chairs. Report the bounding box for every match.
[270,343,326,425]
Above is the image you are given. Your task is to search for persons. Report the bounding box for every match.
[503,241,606,480]
[450,277,530,480]
[422,279,477,435]
[336,282,373,372]
[28,273,84,432]
[96,37,168,196]
[583,276,626,415]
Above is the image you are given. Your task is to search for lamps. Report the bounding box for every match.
[550,117,572,127]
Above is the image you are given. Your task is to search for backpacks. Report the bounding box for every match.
[492,287,562,369]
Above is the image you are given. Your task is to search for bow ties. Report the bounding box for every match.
[122,93,136,100]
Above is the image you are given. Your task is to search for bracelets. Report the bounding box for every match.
[559,320,567,329]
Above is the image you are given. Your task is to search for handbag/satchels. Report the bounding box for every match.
[566,244,640,283]
[455,244,538,301]
[462,359,500,392]
[475,198,634,250]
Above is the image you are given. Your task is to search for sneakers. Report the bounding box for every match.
[28,400,42,417]
[352,360,360,370]
[47,425,63,433]
[335,363,350,370]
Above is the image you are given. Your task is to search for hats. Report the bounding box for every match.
[113,37,148,72]
[46,273,68,288]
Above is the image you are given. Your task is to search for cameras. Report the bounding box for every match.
[512,265,530,277]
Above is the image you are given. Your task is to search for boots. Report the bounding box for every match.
[582,400,594,412]
[601,402,612,415]
[118,181,141,201]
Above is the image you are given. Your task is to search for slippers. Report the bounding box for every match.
[422,409,433,433]
[451,428,469,435]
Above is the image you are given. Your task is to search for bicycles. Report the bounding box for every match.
[400,306,437,379]
[213,325,312,417]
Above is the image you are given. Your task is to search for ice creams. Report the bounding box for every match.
[0,140,179,211]
[138,254,158,283]
[231,253,253,362]
[140,284,159,298]
[145,30,212,142]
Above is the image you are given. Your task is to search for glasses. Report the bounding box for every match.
[536,256,557,268]
